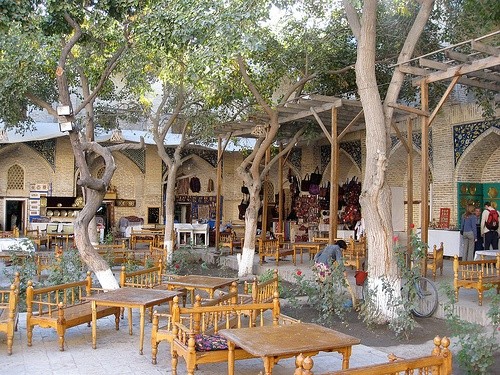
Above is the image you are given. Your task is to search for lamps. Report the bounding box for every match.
[56,105,73,117]
[60,121,73,132]
[251,124,271,138]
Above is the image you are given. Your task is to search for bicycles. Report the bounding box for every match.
[361,251,438,317]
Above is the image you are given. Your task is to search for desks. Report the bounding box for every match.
[164,275,239,316]
[80,286,185,356]
[474,249,500,262]
[120,216,143,238]
[315,238,342,257]
[130,233,160,249]
[48,232,77,252]
[417,228,467,258]
[218,322,361,375]
[293,241,328,265]
[337,230,355,240]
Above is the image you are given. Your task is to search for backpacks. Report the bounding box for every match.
[485,209,499,230]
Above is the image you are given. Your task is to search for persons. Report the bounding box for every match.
[314,240,348,313]
[461,204,484,267]
[481,201,500,268]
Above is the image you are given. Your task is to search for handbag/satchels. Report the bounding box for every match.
[310,166,322,185]
[238,199,249,220]
[309,185,319,194]
[319,176,361,230]
[301,174,310,191]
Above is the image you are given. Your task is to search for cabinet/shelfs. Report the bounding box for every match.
[271,209,360,244]
[40,196,109,244]
[173,223,211,248]
[164,195,223,228]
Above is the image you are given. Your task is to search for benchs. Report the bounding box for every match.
[151,269,282,375]
[26,271,120,351]
[129,229,177,251]
[95,244,188,320]
[453,253,500,306]
[0,225,64,280]
[292,335,452,375]
[0,271,20,355]
[218,228,296,266]
[417,242,443,282]
[342,233,367,272]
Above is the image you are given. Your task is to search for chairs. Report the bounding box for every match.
[115,232,129,249]
